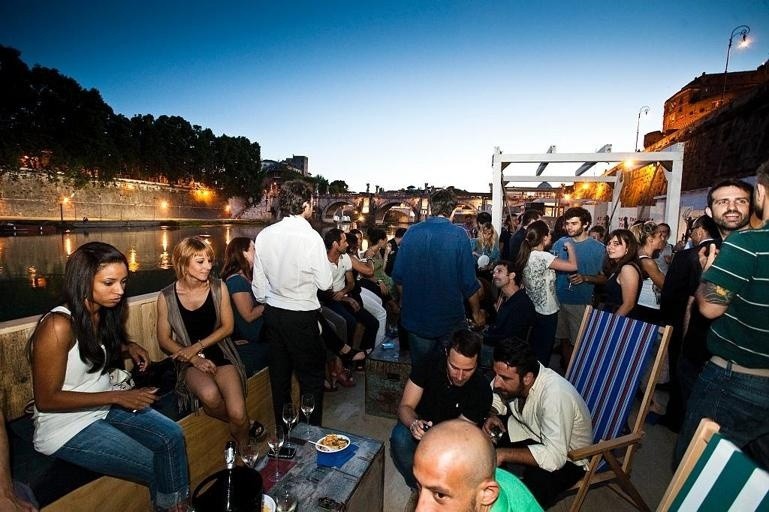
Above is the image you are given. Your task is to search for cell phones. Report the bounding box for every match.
[268,447,296,458]
[423,423,430,431]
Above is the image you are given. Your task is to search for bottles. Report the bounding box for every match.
[219,441,240,511]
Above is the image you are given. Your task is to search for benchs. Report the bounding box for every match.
[0,291,367,512]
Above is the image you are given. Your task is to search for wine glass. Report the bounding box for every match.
[564,271,578,292]
[274,483,299,511]
[299,392,318,440]
[261,424,289,483]
[281,402,300,441]
[235,435,259,469]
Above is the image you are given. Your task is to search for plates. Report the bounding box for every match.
[359,258,375,264]
[316,433,350,454]
[261,492,275,512]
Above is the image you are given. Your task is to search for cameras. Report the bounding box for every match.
[643,223,657,235]
[490,427,502,445]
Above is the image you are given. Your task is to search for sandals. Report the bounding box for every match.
[323,347,373,392]
[249,420,265,437]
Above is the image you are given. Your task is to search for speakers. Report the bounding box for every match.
[526,203,544,216]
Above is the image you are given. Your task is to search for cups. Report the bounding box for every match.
[482,420,507,445]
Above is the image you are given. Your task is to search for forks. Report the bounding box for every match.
[306,440,335,451]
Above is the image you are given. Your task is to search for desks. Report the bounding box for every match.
[254,421,385,512]
[365,326,414,419]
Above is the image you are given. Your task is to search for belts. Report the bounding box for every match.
[710,356,768,377]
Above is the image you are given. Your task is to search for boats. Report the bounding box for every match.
[0,231,60,236]
[0,220,74,231]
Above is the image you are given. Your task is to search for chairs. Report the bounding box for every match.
[563,303,674,512]
[656,416,768,512]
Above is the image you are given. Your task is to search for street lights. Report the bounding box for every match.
[97,192,102,219]
[61,233,66,256]
[721,24,751,105]
[635,105,651,152]
[119,194,123,220]
[59,197,68,221]
[153,196,156,219]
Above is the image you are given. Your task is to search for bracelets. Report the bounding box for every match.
[196,339,204,352]
[410,420,418,432]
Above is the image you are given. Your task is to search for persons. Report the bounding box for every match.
[25,241,194,512]
[157,235,268,466]
[467,206,723,432]
[319,228,408,392]
[389,330,495,497]
[413,419,545,512]
[481,337,593,503]
[391,189,486,359]
[661,163,769,472]
[219,236,264,359]
[252,181,334,434]
